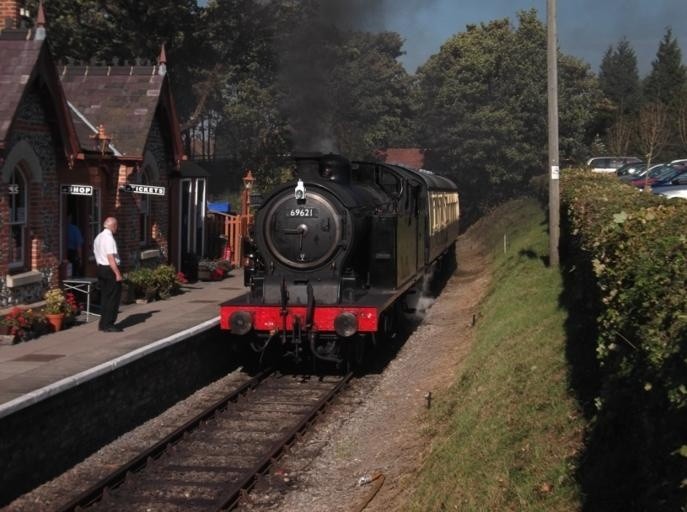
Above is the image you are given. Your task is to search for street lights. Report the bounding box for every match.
[243,171,257,226]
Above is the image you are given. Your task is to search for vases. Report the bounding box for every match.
[44,312,66,331]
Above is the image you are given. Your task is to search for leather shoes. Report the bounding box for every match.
[99,324,122,332]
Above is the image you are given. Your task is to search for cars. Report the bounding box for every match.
[558,156,574,169]
[583,153,687,204]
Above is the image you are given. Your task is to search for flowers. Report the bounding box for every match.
[65,293,84,322]
[1,307,36,336]
[43,288,67,314]
[176,272,187,284]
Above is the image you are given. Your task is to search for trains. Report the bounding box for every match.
[218,148,463,374]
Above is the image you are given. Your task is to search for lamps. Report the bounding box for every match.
[88,125,112,159]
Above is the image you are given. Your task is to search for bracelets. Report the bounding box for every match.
[78,257,82,260]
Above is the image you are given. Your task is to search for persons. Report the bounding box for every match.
[66,212,83,276]
[93,216,124,332]
